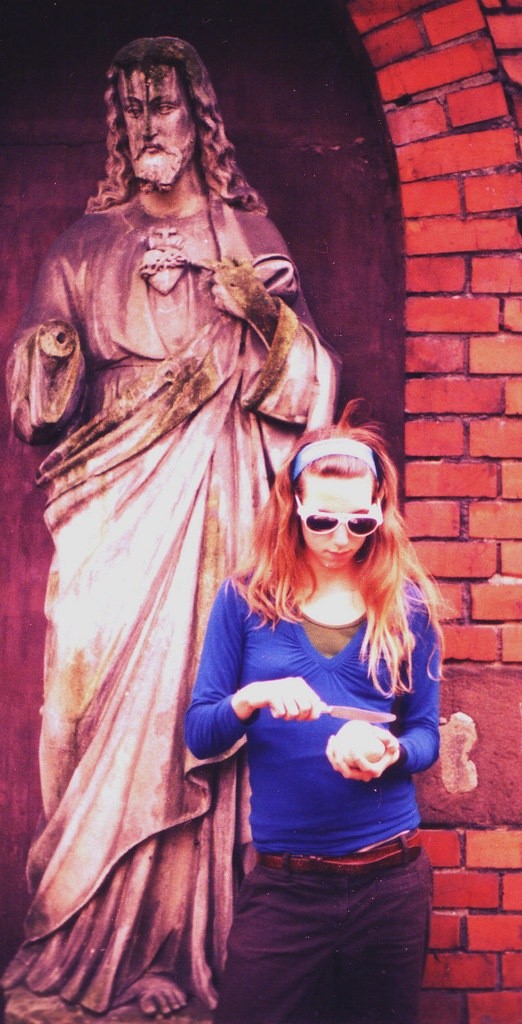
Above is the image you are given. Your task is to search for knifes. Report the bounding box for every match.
[321,704,397,725]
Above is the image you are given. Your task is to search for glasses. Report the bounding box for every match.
[294,492,383,537]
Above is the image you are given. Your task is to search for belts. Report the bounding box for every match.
[258,829,422,875]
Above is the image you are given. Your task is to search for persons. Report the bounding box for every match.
[1,33,345,1014]
[184,421,444,1024]
